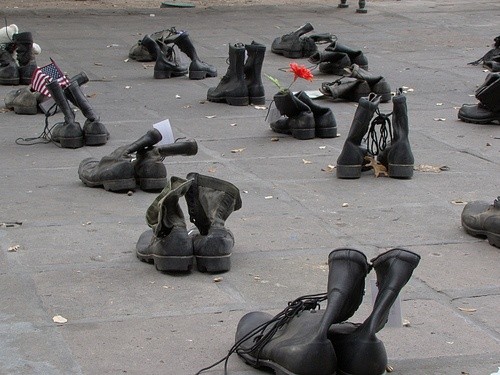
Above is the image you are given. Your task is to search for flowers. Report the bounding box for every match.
[266,62,313,93]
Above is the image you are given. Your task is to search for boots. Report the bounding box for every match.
[271,22,368,76]
[45,80,83,148]
[0,43,20,85]
[64,81,109,145]
[377,95,414,177]
[483,36,500,72]
[173,31,217,79]
[294,90,337,138]
[458,74,500,124]
[135,176,196,273]
[207,42,249,106]
[13,32,41,84]
[343,63,391,101]
[327,249,421,375]
[133,137,197,190]
[78,128,162,192]
[244,40,266,104]
[35,71,89,115]
[4,84,41,114]
[336,93,382,178]
[236,247,372,375]
[270,89,315,140]
[319,76,371,101]
[142,35,188,79]
[185,173,241,273]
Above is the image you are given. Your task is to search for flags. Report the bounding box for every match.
[30,60,69,96]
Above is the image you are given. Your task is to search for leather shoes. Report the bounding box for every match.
[462,196,500,248]
